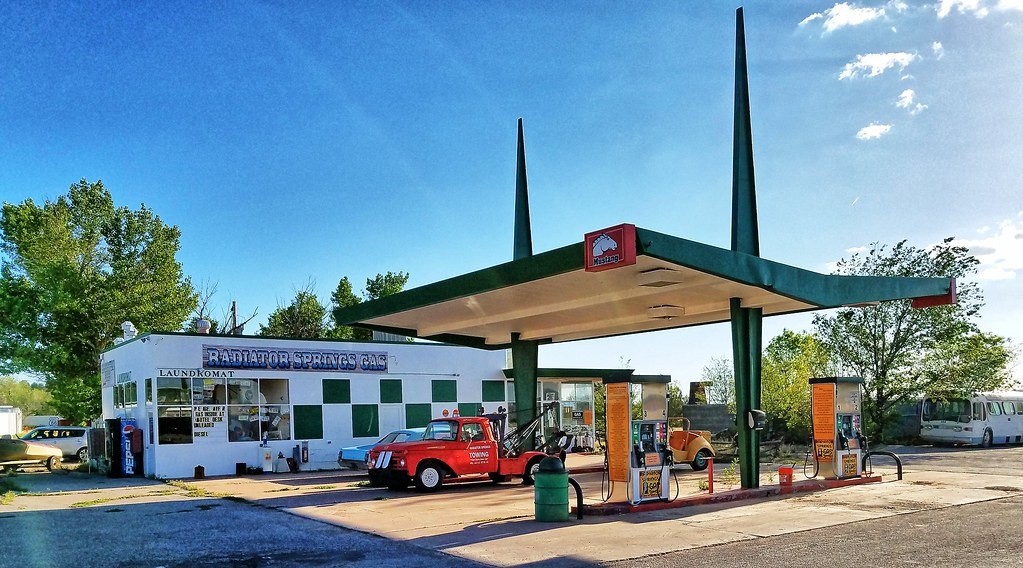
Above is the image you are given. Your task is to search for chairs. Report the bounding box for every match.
[670,430,686,451]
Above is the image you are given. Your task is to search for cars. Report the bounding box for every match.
[337,427,469,470]
[665,416,715,470]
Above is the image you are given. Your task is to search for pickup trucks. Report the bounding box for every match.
[366,397,574,493]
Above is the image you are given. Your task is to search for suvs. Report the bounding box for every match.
[19,427,92,462]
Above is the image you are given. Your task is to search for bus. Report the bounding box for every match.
[916,387,1023,448]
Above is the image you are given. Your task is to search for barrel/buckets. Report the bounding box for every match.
[778,467,793,485]
[534,470,569,521]
